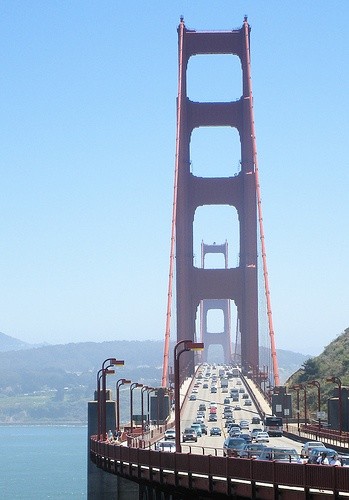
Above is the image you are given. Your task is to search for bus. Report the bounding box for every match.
[265,416,283,437]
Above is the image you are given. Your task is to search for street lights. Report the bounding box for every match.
[173,340,204,453]
[291,384,308,432]
[96,358,170,441]
[326,376,342,436]
[305,380,320,431]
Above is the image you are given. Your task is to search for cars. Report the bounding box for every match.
[164,363,339,466]
